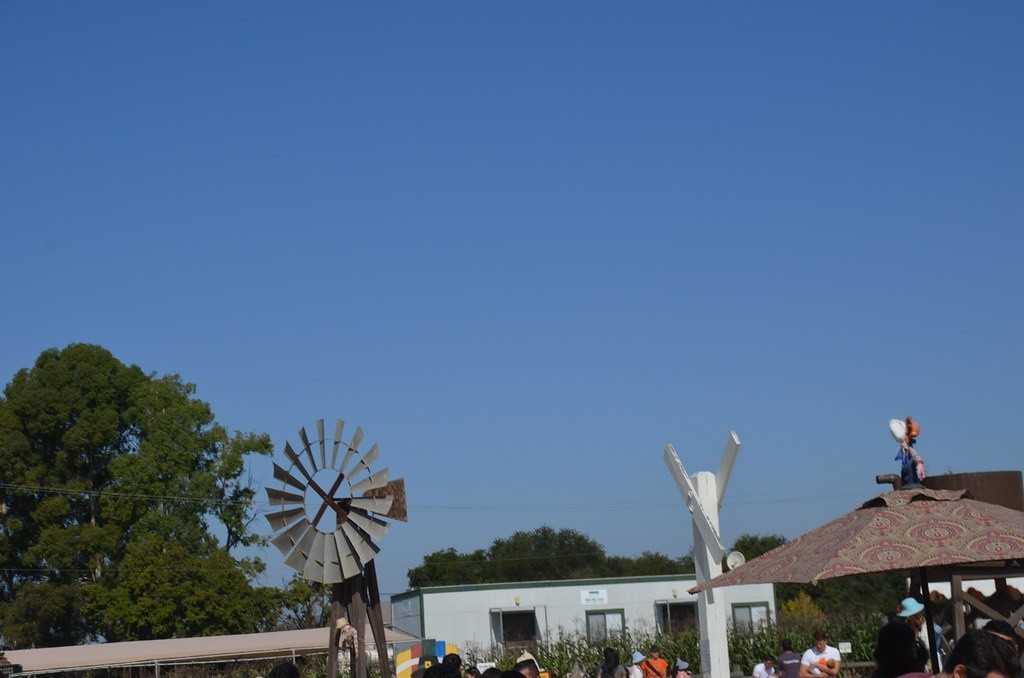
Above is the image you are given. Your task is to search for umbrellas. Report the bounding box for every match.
[687,483,1024,675]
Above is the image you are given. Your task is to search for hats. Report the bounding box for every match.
[631,652,647,664]
[650,645,660,652]
[983,620,1015,637]
[672,659,689,669]
[896,597,924,616]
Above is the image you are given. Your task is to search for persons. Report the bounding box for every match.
[874,586,1024,678]
[569,645,689,678]
[271,653,540,678]
[751,629,841,678]
